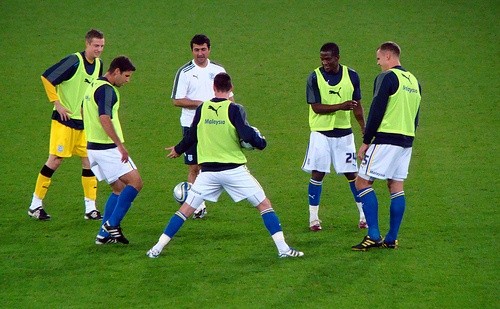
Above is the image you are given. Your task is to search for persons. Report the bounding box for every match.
[300,42,369,231]
[80,56,144,245]
[351,41,422,251]
[145,73,305,258]
[170,33,236,220]
[27,29,105,221]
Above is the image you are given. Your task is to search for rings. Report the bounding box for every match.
[172,155,175,157]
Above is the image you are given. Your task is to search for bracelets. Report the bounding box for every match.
[360,125,366,129]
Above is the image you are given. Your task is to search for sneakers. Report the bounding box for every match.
[192,210,205,219]
[202,207,208,214]
[101,220,129,244]
[351,235,384,251]
[84,210,104,220]
[96,234,120,244]
[28,206,51,220]
[278,247,304,258]
[378,239,399,249]
[310,221,322,231]
[359,219,368,229]
[145,249,160,258]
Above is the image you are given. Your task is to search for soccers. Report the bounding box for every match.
[173,182,194,203]
[238,125,261,149]
